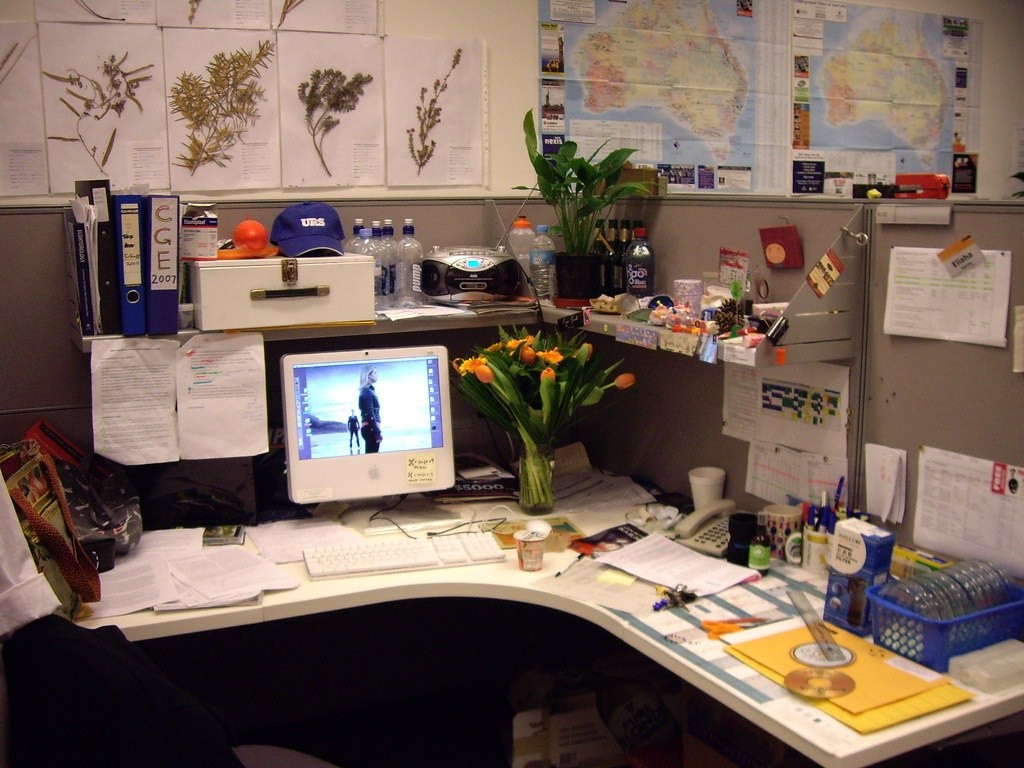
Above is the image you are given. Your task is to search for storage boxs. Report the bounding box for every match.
[948,638,1023,695]
[822,517,895,637]
[184,252,375,334]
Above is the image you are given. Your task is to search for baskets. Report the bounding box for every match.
[866,576,1024,672]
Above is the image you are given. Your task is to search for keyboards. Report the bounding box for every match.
[299,531,509,581]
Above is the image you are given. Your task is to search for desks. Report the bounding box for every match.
[73,495,1024,768]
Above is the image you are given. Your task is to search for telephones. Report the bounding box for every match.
[675,498,758,557]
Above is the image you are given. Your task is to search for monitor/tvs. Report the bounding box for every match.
[280,345,464,532]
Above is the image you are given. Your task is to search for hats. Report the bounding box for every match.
[270,200,345,257]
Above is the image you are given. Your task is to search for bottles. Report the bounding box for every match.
[530,225,554,302]
[509,215,536,282]
[346,219,397,311]
[749,524,770,575]
[591,217,643,298]
[394,219,424,305]
[625,228,654,299]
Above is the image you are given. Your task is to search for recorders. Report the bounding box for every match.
[419,183,539,304]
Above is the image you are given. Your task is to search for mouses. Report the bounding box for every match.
[525,519,552,534]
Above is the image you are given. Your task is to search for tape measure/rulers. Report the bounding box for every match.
[785,587,847,662]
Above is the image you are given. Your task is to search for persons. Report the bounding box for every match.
[357,363,384,456]
[347,409,361,450]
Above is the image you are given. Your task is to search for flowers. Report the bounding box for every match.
[452,322,639,444]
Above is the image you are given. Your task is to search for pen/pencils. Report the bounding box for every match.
[555,552,585,577]
[805,476,846,532]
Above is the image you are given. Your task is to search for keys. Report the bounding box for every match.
[665,585,696,611]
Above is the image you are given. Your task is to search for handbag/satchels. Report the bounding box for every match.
[1,443,100,628]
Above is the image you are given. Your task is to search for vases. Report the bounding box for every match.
[513,441,566,520]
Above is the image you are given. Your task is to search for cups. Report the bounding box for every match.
[688,466,725,513]
[756,503,803,560]
[785,526,832,574]
[726,512,758,565]
[513,530,546,572]
[673,279,702,318]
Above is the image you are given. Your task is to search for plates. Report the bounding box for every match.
[882,554,1021,661]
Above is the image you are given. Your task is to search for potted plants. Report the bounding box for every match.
[522,107,654,301]
